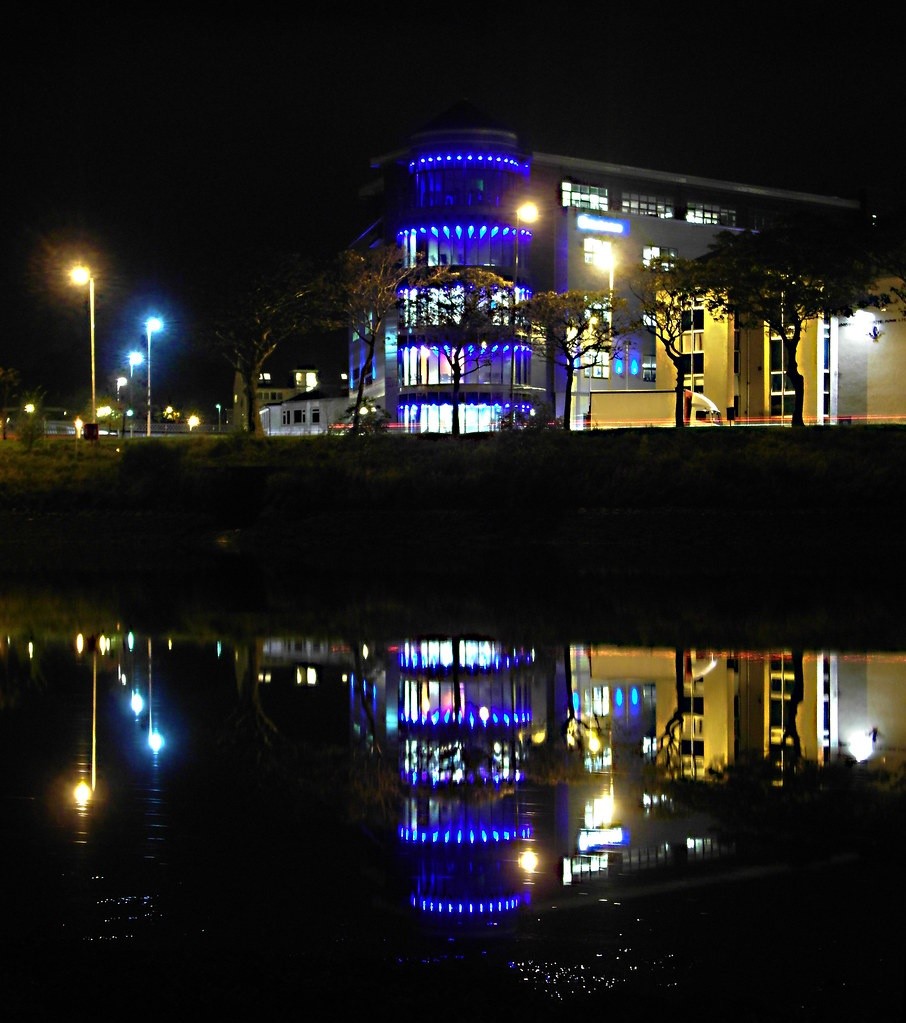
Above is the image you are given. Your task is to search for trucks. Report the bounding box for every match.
[582,388,725,430]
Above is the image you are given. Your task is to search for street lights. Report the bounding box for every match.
[3,265,200,439]
[2,627,170,815]
